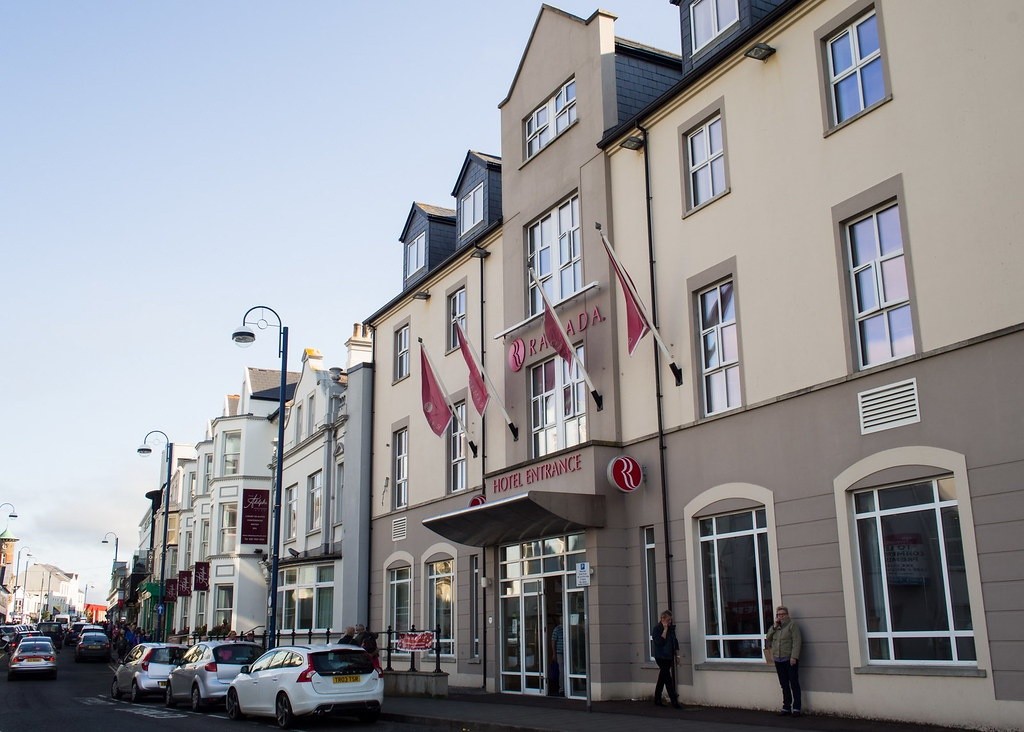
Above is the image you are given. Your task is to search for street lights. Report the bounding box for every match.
[12,546,38,624]
[137,430,172,642]
[84,583,96,611]
[231,305,287,652]
[102,532,118,630]
[46,568,60,616]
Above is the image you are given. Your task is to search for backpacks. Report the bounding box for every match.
[361,631,377,653]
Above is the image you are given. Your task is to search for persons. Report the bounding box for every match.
[95,621,255,659]
[338,625,378,668]
[552,617,565,695]
[651,610,682,709]
[766,607,802,718]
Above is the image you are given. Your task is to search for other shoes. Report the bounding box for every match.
[792,711,800,717]
[776,710,792,716]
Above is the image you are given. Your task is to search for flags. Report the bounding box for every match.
[530,269,573,364]
[420,344,454,437]
[601,237,651,358]
[455,317,489,417]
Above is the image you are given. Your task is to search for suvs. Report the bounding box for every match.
[12,630,44,651]
[36,622,63,650]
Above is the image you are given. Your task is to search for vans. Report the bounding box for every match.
[54,614,70,630]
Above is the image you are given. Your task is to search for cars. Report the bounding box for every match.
[225,644,384,729]
[8,636,59,679]
[165,641,264,713]
[64,622,105,646]
[0,625,37,653]
[112,642,189,703]
[75,632,111,661]
